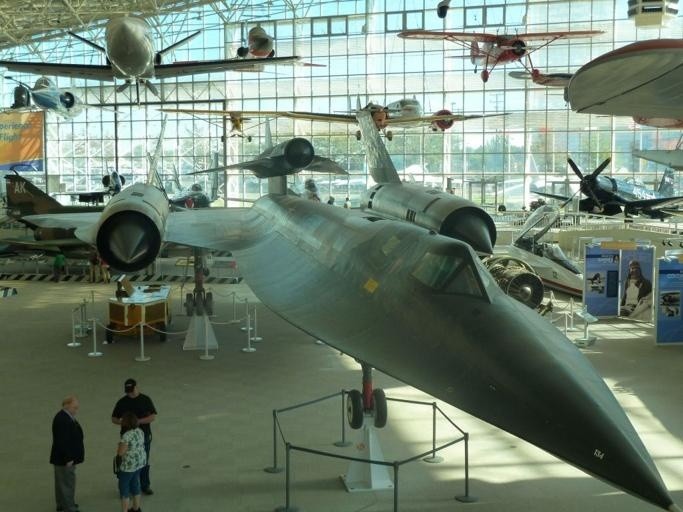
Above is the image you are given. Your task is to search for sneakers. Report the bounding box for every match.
[128,507,141,512]
[142,488,154,495]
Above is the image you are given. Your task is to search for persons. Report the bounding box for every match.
[116,410,148,512]
[52,244,111,283]
[619,260,652,317]
[111,377,158,501]
[48,394,85,512]
[184,196,195,208]
[312,193,352,208]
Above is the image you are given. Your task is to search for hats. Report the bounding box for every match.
[628,260,639,268]
[124,379,136,393]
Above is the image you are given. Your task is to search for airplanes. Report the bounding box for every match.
[1,17,302,82]
[228,27,326,74]
[528,157,683,224]
[533,38,682,130]
[400,27,603,81]
[20,109,683,512]
[292,100,511,140]
[1,74,122,121]
